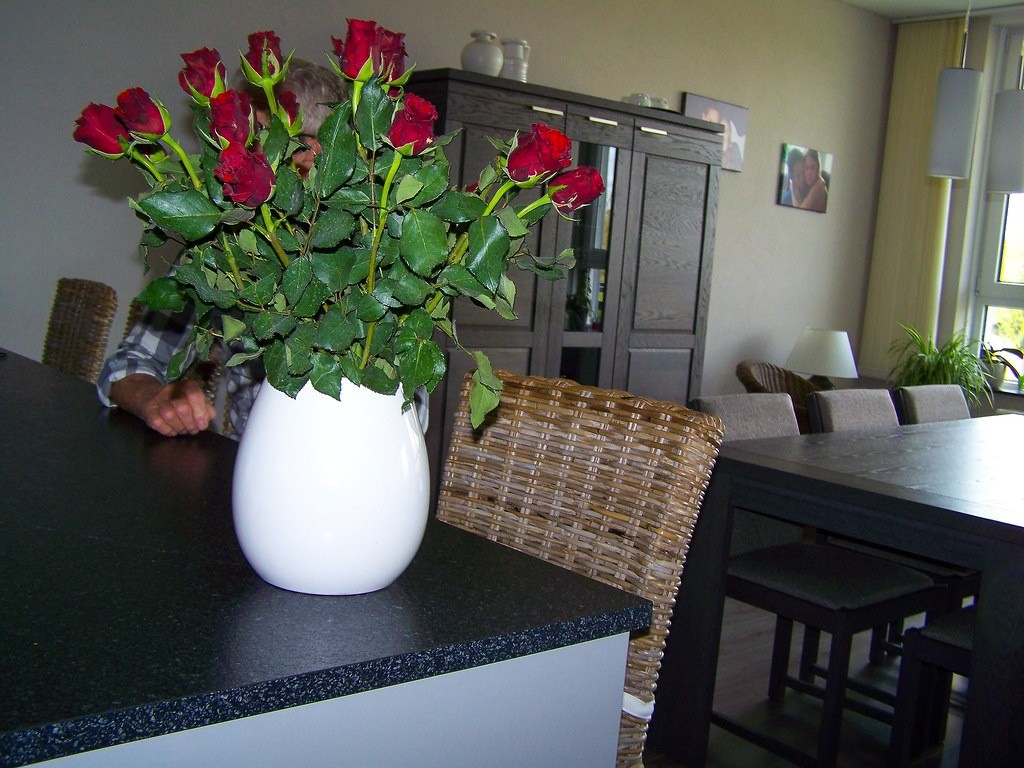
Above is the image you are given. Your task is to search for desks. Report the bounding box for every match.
[644,415,1024,768]
[0,343,656,767]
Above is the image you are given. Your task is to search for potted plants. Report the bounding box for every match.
[980,342,1024,389]
[589,311,603,332]
[566,293,592,332]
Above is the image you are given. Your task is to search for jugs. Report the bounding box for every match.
[500,38,530,83]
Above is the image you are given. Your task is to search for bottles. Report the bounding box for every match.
[460,29,503,76]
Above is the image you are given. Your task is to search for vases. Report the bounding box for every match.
[460,31,504,77]
[231,375,431,596]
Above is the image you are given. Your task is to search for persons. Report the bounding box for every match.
[96,56,429,437]
[702,104,741,171]
[783,149,827,212]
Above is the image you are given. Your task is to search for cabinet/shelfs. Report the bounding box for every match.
[400,67,724,518]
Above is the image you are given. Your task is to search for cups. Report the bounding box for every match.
[628,92,652,108]
[649,96,669,109]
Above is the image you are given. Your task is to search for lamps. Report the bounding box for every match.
[924,32,984,180]
[986,56,1024,194]
[784,328,859,390]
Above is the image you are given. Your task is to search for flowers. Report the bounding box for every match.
[73,18,605,432]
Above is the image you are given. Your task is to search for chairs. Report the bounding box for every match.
[736,359,823,435]
[436,369,727,767]
[688,382,980,768]
[42,277,118,384]
